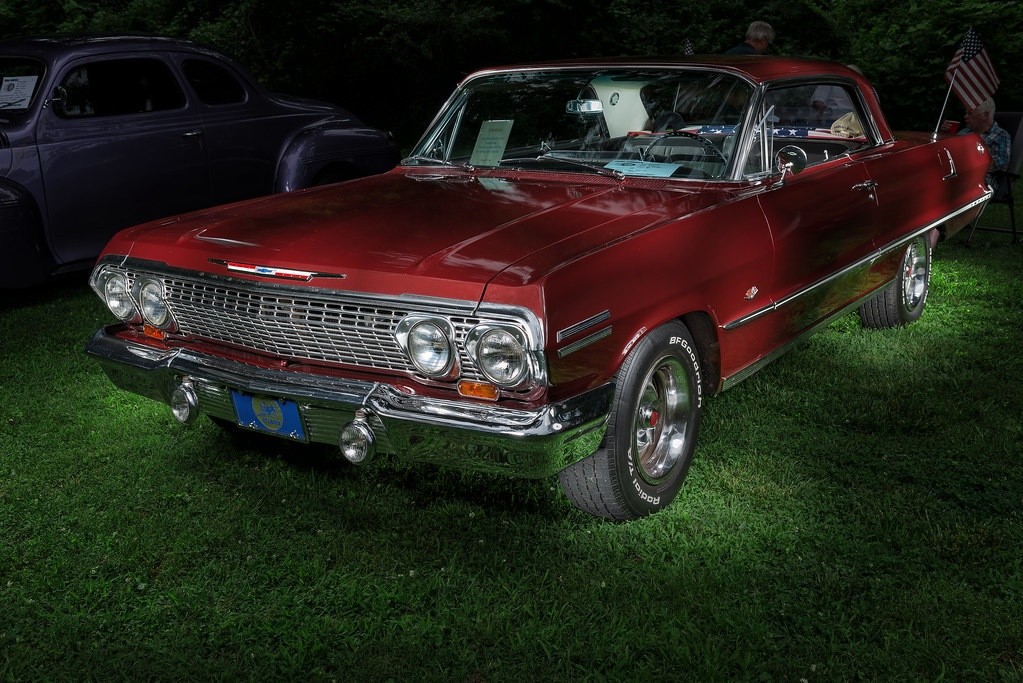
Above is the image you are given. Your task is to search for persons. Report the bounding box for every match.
[810,66,880,129]
[724,22,774,55]
[942,96,1010,195]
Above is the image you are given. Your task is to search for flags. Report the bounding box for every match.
[945,26,999,111]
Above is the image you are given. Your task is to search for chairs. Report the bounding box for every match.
[958,111,1023,246]
[639,146,830,167]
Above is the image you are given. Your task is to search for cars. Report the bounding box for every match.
[83,54,993,524]
[0,35,393,297]
